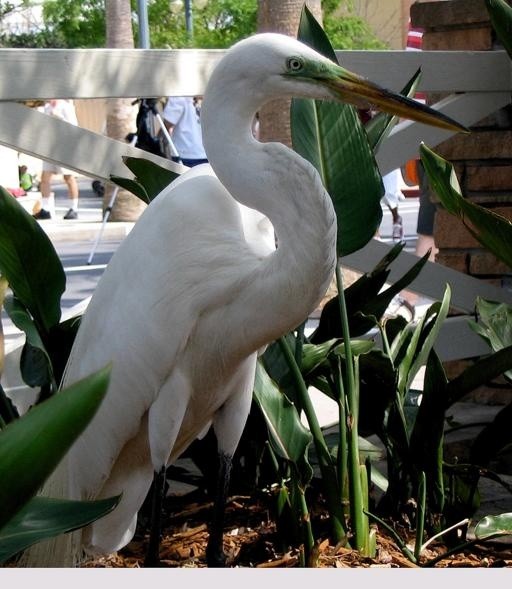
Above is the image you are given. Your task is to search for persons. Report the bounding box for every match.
[19,96,209,220]
[254,112,260,141]
[393,18,436,322]
[358,106,406,244]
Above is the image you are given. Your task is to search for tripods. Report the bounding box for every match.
[87,98,184,265]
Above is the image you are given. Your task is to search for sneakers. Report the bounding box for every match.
[63,208,78,220]
[32,209,51,220]
[392,216,404,244]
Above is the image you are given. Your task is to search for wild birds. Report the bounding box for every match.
[11,32,473,568]
